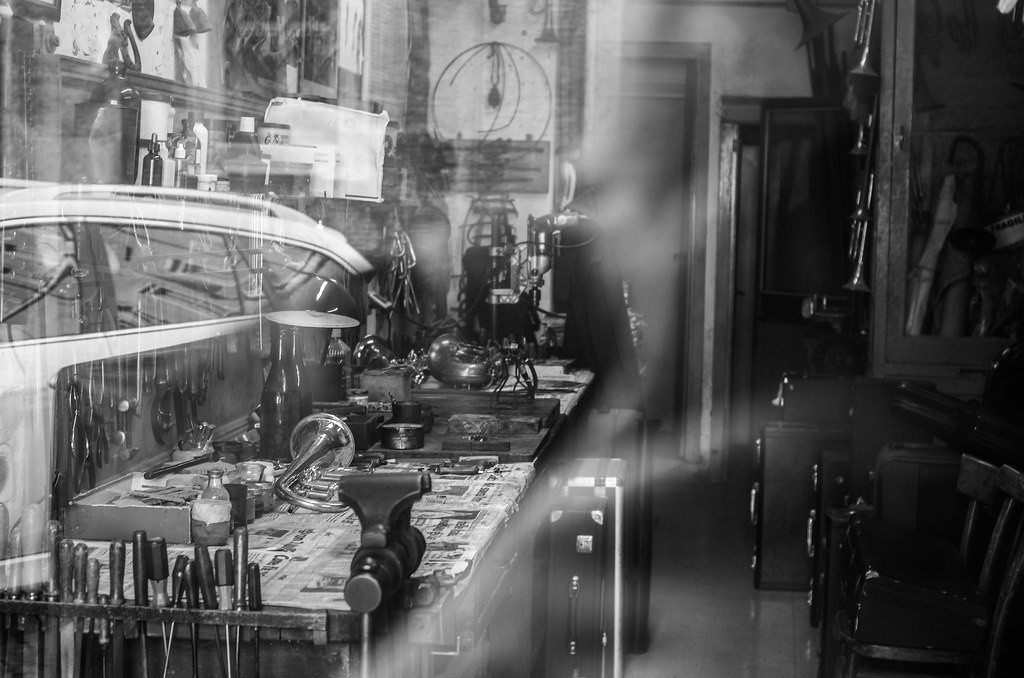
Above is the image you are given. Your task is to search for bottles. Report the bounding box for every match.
[262,330,299,461]
[90,60,141,184]
[202,469,230,502]
[325,329,351,394]
[289,330,313,419]
[142,110,261,194]
[248,486,264,517]
[254,482,273,513]
[246,492,255,524]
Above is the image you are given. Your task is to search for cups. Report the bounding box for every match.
[213,442,242,462]
[183,441,207,452]
[223,484,247,526]
[393,401,421,426]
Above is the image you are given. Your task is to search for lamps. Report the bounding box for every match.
[265,272,360,329]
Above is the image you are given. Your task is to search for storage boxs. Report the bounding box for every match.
[62,472,209,545]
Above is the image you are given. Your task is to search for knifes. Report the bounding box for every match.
[0,504,75,678]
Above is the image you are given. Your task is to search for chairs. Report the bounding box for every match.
[841,453,1024,678]
